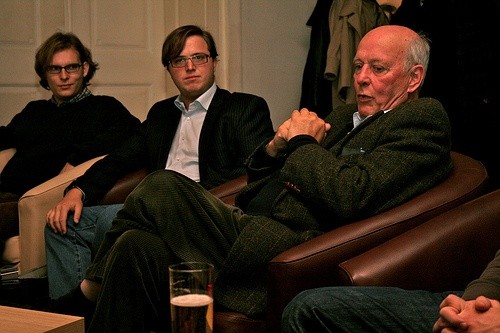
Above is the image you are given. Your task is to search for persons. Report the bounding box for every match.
[0,33,141,266]
[282,247,500,333]
[48,24,452,333]
[44,25,274,300]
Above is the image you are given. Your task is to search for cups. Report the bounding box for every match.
[168,261,215,333]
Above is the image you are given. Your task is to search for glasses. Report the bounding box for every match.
[45,62,84,74]
[168,53,212,68]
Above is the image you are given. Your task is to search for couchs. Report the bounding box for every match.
[208,150,488,333]
[0,148,109,278]
[334,188,500,292]
